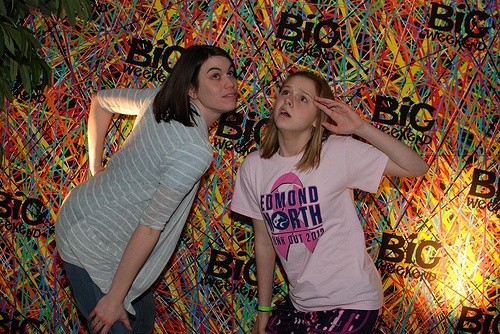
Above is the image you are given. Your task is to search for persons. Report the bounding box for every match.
[54,44,238,334]
[230,70,430,334]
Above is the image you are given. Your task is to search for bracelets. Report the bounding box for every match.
[257,305,276,312]
